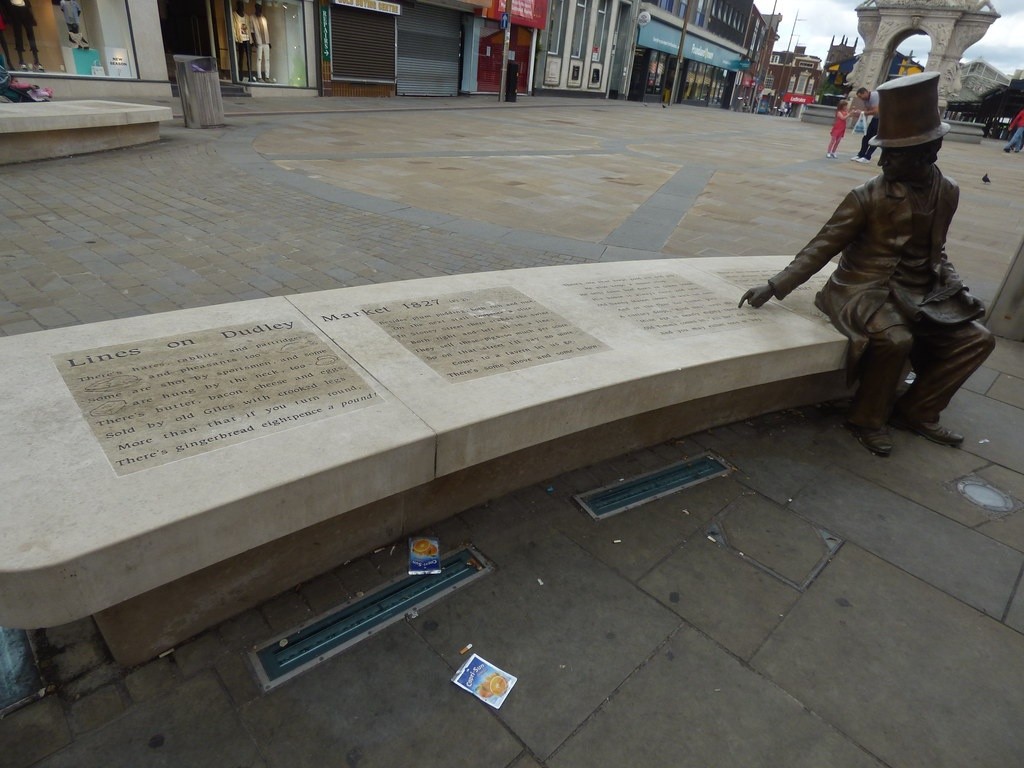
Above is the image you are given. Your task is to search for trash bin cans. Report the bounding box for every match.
[172,55,226,130]
[506,63,519,102]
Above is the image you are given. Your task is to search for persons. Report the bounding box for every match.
[250,3,272,82]
[233,0,255,82]
[69,23,89,50]
[851,87,879,163]
[779,105,792,117]
[9,76,54,102]
[1004,108,1024,153]
[60,0,81,43]
[738,71,997,456]
[826,100,855,158]
[0,0,44,71]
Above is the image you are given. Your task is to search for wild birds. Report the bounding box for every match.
[662,104,666,108]
[981,173,991,184]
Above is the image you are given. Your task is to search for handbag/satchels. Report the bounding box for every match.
[852,111,867,136]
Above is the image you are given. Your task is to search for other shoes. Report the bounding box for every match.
[857,157,869,163]
[258,79,265,82]
[826,152,831,158]
[1003,148,1010,153]
[19,63,27,69]
[850,156,859,161]
[33,64,43,72]
[1015,149,1019,153]
[265,79,274,82]
[831,153,837,158]
